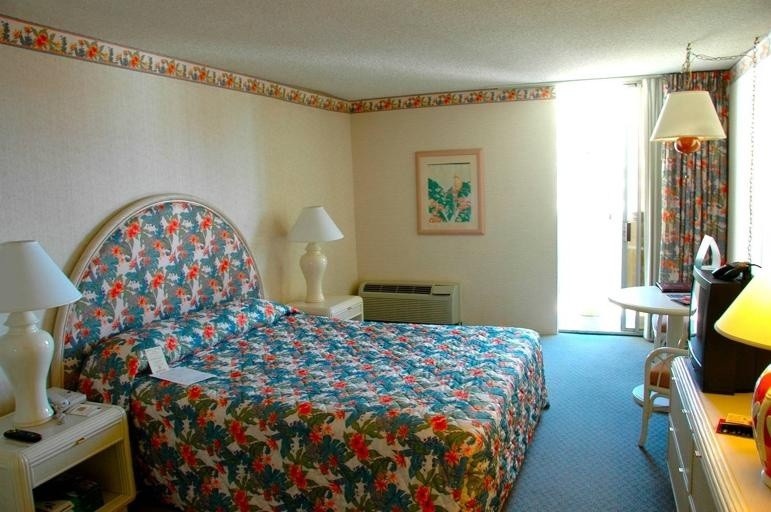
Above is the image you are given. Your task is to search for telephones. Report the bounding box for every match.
[47,387,87,412]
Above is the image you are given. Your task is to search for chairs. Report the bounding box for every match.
[638,334,690,448]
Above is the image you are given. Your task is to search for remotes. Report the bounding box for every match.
[4,429,41,443]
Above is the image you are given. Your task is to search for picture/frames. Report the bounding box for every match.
[414,149,485,236]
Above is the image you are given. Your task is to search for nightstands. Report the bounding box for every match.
[0,400,137,512]
[286,294,364,321]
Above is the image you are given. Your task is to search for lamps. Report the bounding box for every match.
[1,240,84,429]
[649,41,726,156]
[714,272,771,490]
[286,206,343,303]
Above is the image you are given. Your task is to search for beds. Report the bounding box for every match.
[51,192,550,512]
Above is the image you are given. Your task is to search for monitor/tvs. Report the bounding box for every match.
[688,264,771,395]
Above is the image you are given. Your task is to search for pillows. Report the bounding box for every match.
[83,297,290,384]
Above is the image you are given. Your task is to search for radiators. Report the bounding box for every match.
[358,279,460,325]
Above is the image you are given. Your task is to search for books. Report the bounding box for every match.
[35,500,74,512]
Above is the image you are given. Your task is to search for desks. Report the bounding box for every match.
[607,286,690,414]
[666,354,771,512]
